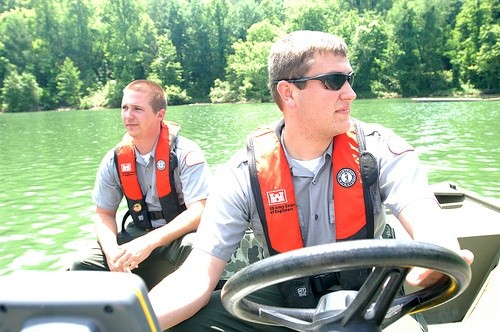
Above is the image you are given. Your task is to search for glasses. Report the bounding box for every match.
[273,70,355,91]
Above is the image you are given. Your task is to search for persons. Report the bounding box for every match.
[146,30,475,332]
[62,79,212,271]
